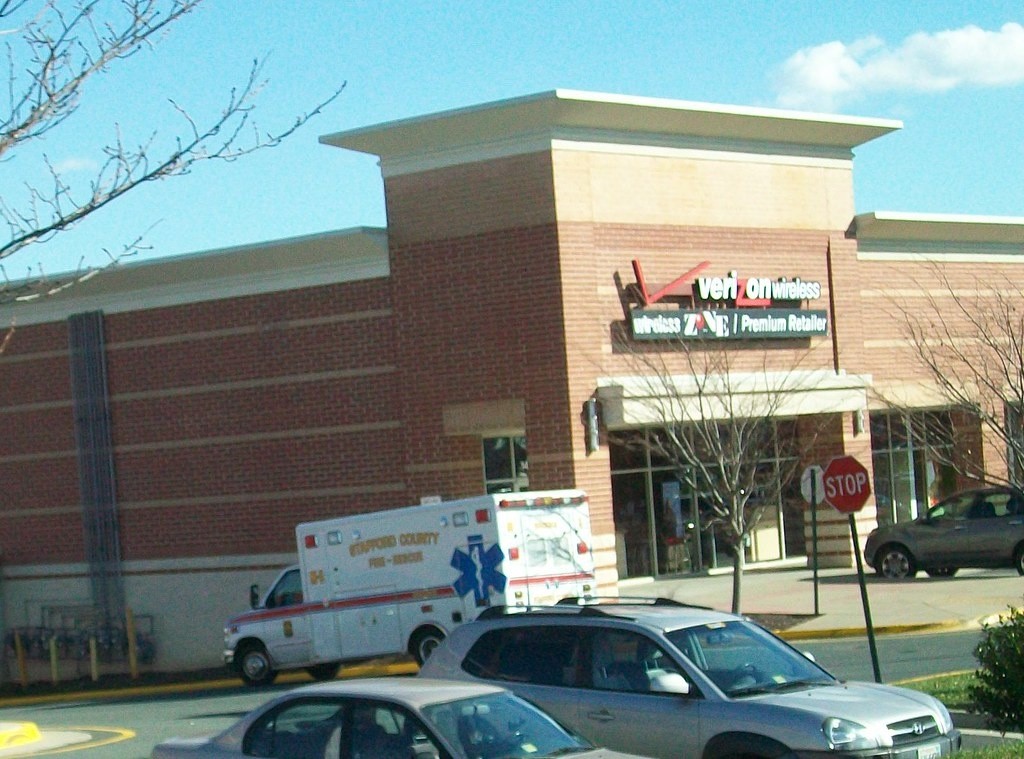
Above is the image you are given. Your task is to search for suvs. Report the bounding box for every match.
[412,595,964,759]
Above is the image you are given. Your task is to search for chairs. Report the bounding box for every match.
[638,643,676,691]
[354,725,399,759]
[581,635,633,690]
[400,717,440,759]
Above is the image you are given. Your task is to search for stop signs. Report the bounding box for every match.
[824,455,872,517]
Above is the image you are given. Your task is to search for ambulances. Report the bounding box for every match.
[223,489,598,688]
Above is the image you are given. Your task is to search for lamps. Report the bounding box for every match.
[855,410,865,434]
[586,397,599,453]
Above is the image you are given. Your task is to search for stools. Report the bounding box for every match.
[665,537,692,576]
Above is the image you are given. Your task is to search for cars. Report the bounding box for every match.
[151,678,650,759]
[864,485,1024,579]
